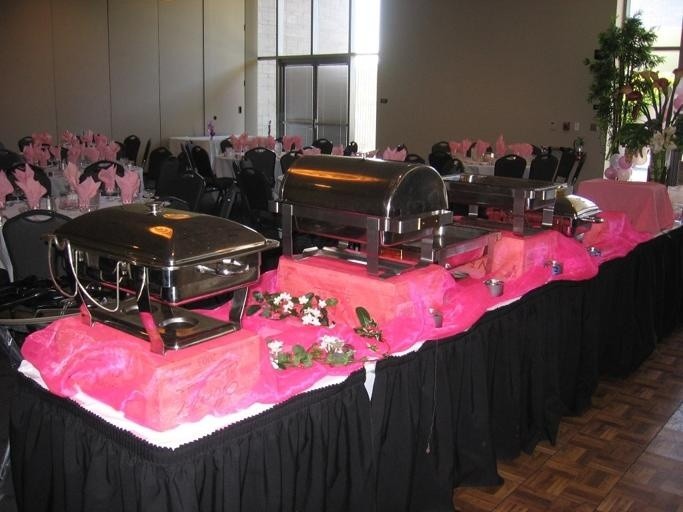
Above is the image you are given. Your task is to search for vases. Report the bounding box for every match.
[649,148,680,186]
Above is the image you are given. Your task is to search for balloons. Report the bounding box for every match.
[605,145,648,181]
[662,78,683,122]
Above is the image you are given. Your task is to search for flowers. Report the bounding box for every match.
[615,66,682,152]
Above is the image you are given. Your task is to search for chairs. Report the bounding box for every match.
[0,133,591,344]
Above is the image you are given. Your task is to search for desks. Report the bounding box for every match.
[0,219,682,512]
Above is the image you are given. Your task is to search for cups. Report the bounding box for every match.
[24,157,136,171]
[450,141,534,165]
[11,183,139,214]
[225,141,282,158]
